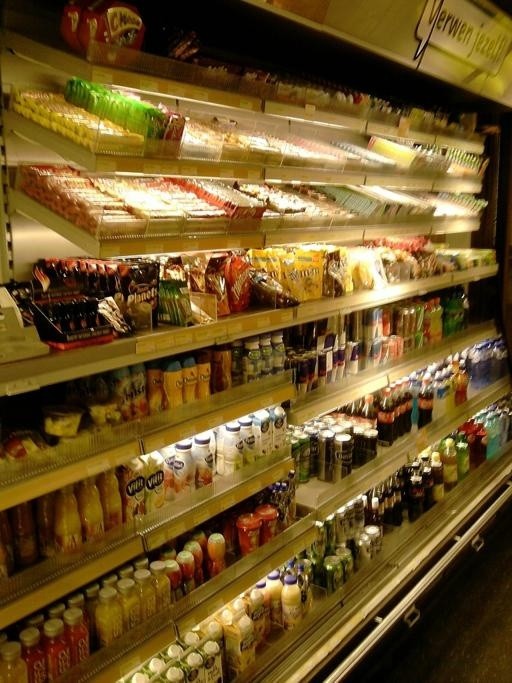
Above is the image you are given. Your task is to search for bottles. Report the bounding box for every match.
[2,330,316,682]
[339,292,512,531]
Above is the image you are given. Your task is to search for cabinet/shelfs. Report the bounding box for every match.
[0,29,511,682]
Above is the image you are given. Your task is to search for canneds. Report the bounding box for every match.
[285,416,379,482]
[295,494,383,596]
